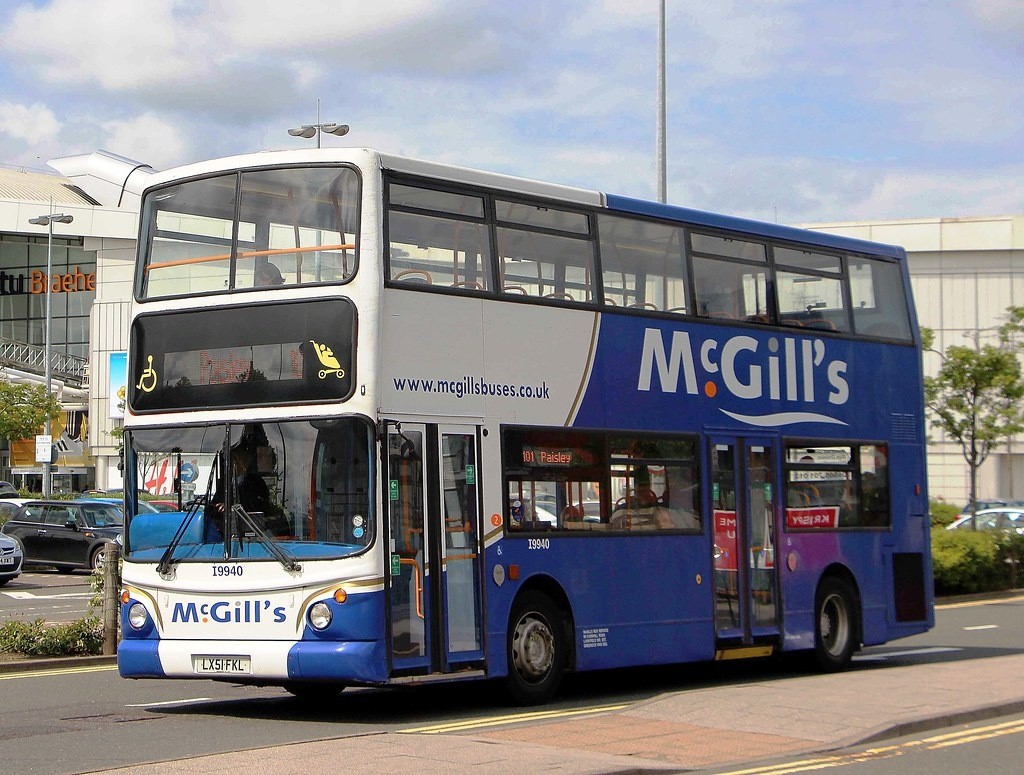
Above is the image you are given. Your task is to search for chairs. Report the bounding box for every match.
[391,267,905,350]
[508,475,870,530]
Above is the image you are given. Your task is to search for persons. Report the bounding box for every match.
[862,471,888,527]
[209,449,270,542]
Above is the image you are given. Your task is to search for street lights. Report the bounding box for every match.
[27,194,74,501]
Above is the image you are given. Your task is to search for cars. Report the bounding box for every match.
[955,501,1024,524]
[148,500,200,514]
[2,499,133,576]
[0,530,23,586]
[77,497,159,520]
[945,508,1024,537]
[506,485,640,532]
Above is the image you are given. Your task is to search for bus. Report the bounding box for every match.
[116,145,937,690]
